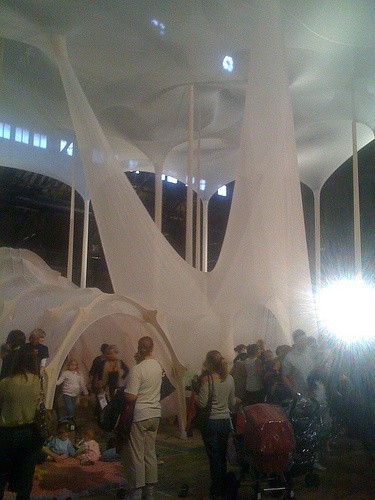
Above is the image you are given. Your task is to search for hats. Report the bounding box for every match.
[289,396,319,418]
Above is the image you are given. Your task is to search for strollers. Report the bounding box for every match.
[226,391,297,500]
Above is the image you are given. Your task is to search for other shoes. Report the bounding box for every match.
[157,457,164,464]
[313,462,326,471]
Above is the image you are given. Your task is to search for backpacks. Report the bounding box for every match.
[228,357,249,400]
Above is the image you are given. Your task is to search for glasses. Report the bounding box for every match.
[105,350,120,355]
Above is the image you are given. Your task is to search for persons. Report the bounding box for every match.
[89,343,129,395]
[41,425,100,466]
[230,330,375,473]
[195,350,237,500]
[56,359,87,418]
[0,329,49,500]
[122,336,163,500]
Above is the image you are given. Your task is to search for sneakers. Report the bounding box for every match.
[142,485,156,500]
[116,489,142,500]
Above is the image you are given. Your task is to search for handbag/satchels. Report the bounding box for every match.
[158,361,176,401]
[97,387,125,434]
[193,373,214,435]
[35,375,47,432]
